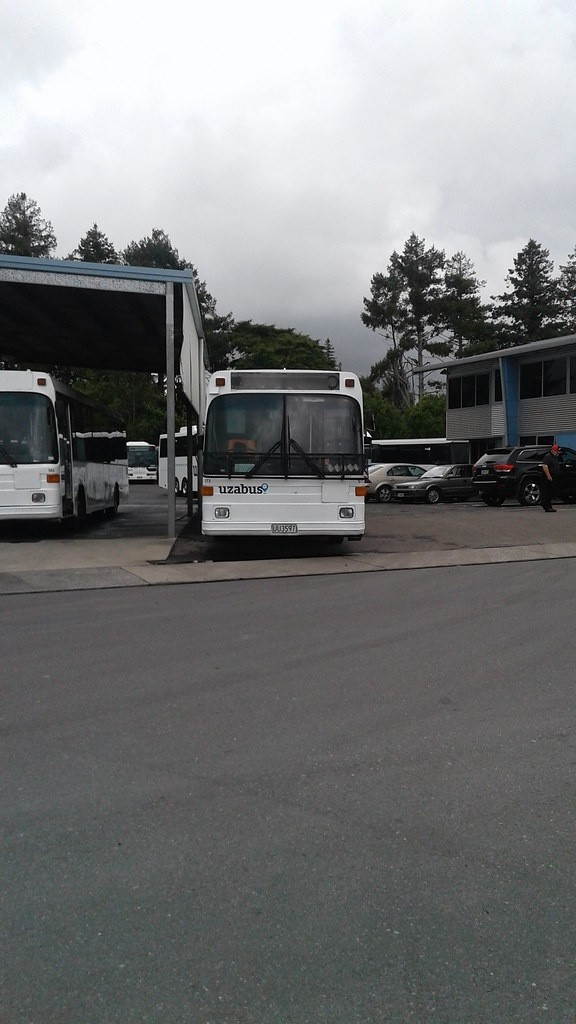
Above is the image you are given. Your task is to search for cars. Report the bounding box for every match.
[363,462,429,502]
[390,463,478,503]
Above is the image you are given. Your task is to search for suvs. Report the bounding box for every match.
[472,444,576,505]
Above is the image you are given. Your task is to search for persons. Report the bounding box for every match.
[539,444,563,512]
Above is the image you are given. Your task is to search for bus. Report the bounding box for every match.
[125,440,158,483]
[157,425,207,500]
[0,360,130,530]
[189,368,373,549]
[363,436,472,475]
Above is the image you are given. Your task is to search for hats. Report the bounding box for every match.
[552,445,562,451]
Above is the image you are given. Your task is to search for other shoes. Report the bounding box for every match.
[545,508,556,512]
